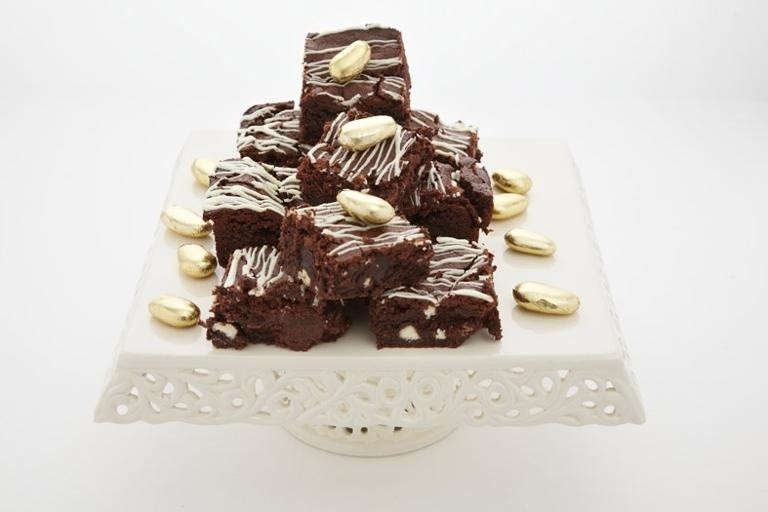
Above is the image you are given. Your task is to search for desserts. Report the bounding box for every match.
[201,25,502,349]
